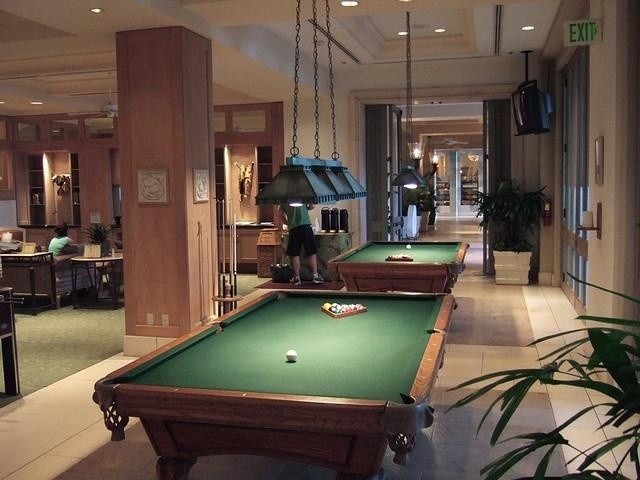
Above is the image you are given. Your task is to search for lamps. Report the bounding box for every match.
[254,0,369,207]
[407,143,424,171]
[431,154,439,172]
[390,12,425,191]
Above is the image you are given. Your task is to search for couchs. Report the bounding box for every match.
[0,251,96,309]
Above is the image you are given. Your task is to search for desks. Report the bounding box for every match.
[281,231,354,282]
[0,251,56,315]
[70,253,123,310]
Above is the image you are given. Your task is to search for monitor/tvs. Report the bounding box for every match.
[511,79,554,136]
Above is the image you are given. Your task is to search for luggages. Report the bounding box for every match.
[270,262,293,284]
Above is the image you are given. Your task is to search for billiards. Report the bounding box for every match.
[388,254,411,260]
[406,244,411,249]
[286,350,298,362]
[322,302,363,315]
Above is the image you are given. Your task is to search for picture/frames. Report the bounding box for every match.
[192,168,210,203]
[136,166,170,206]
[594,136,604,186]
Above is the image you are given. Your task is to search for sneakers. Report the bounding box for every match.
[313,274,324,284]
[289,276,302,286]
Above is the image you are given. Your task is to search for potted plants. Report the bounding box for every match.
[81,222,113,256]
[472,181,548,284]
[405,172,435,232]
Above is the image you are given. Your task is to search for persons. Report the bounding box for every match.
[49,223,78,256]
[278,202,325,285]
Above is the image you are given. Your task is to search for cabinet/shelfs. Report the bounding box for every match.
[12,149,80,229]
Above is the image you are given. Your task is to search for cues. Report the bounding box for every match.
[218,198,238,319]
